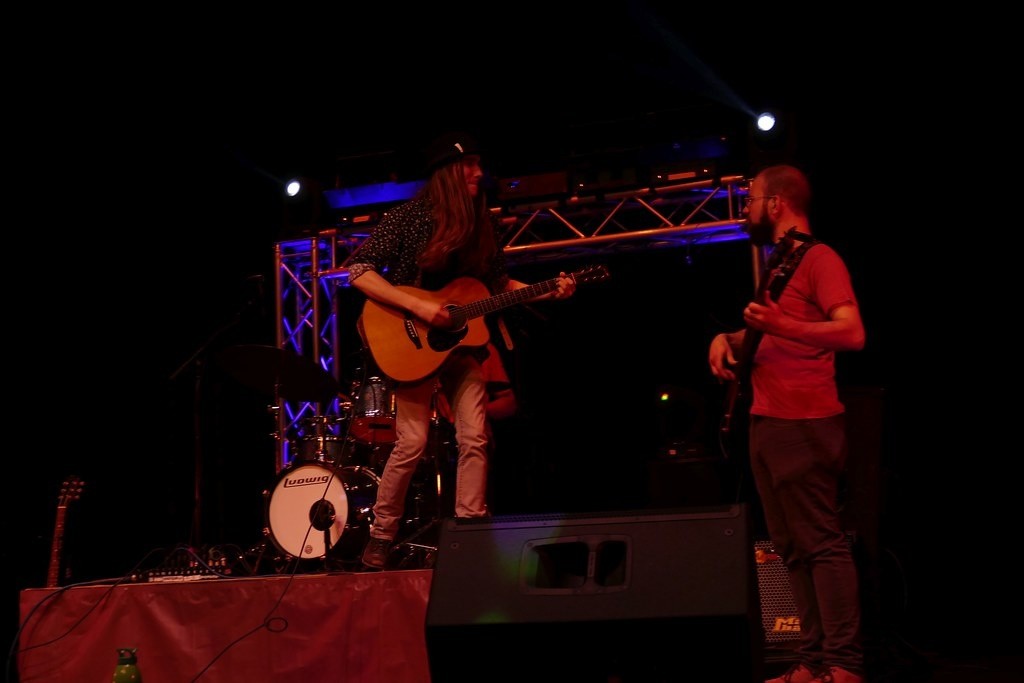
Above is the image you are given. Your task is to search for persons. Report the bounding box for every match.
[706,165,871,683]
[345,139,578,572]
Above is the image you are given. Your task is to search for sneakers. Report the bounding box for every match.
[361,532,392,570]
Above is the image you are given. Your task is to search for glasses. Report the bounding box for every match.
[743,194,776,205]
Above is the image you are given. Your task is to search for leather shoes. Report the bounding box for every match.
[763,662,865,683]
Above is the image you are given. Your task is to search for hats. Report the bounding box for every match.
[426,130,484,170]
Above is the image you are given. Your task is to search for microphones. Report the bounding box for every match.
[247,273,265,302]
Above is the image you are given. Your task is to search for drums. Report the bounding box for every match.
[344,375,403,443]
[338,459,416,540]
[203,345,332,404]
[298,414,350,463]
[263,458,351,560]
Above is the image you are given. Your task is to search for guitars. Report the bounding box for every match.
[46,478,84,588]
[360,266,611,386]
[719,227,795,462]
